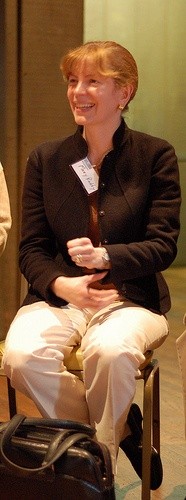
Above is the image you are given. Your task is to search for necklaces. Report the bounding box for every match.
[82,126,113,168]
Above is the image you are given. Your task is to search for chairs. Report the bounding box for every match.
[0,339,160,500]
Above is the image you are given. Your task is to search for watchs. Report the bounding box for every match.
[102,251,111,270]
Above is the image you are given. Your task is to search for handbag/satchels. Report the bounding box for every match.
[0,414,116,500]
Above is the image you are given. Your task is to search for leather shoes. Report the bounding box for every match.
[119,404,163,490]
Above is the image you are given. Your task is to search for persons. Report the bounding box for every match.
[2,41,182,491]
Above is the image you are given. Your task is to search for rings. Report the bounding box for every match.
[76,254,82,263]
